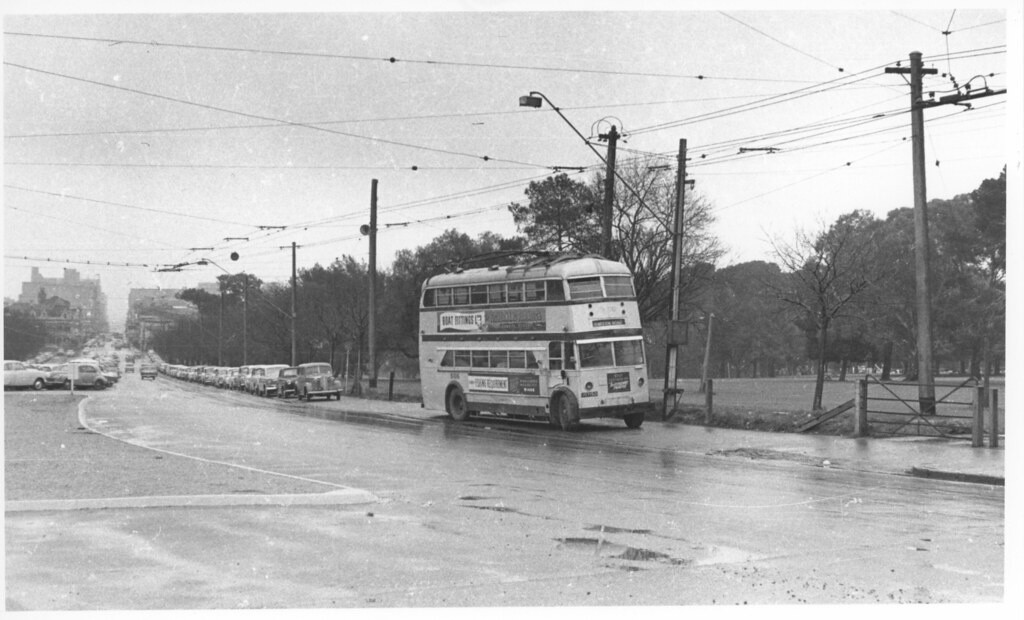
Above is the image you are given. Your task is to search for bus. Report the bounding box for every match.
[418,247,655,429]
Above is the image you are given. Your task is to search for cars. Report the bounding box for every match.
[146,348,344,400]
[139,362,158,381]
[4,332,142,391]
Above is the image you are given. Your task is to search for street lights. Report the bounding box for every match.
[518,90,682,419]
